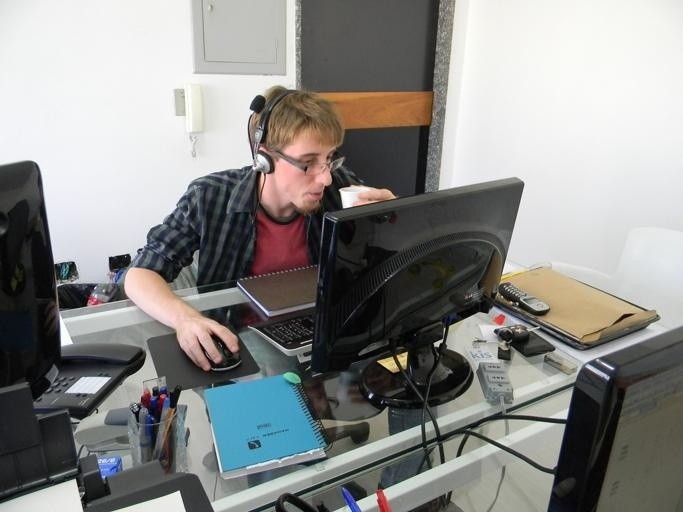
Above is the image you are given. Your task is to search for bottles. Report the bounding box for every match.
[86,271,116,306]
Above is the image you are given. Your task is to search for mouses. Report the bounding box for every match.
[193,322,244,374]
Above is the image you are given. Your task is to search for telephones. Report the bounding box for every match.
[31,340,147,421]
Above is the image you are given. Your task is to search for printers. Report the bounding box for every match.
[1,409,215,512]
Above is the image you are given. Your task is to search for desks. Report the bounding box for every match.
[0,263,672,511]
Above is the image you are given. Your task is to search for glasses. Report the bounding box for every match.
[262,146,345,176]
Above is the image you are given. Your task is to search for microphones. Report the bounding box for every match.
[242,95,267,161]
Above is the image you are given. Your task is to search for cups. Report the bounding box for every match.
[339,187,371,210]
[127,404,181,468]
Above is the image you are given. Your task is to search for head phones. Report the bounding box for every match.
[251,89,332,175]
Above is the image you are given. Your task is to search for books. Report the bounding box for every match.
[203,372,331,479]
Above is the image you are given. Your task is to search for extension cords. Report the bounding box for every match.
[476,360,514,406]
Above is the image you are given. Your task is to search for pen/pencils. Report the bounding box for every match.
[375,488,390,512]
[128,383,185,473]
[340,485,360,512]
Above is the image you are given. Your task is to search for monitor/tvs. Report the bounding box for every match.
[311,176,525,407]
[0,160,62,400]
[547,325,683,511]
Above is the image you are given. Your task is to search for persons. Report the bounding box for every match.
[119,86,397,373]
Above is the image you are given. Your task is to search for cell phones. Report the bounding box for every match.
[498,280,550,317]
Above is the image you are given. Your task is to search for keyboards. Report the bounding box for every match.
[248,314,314,364]
